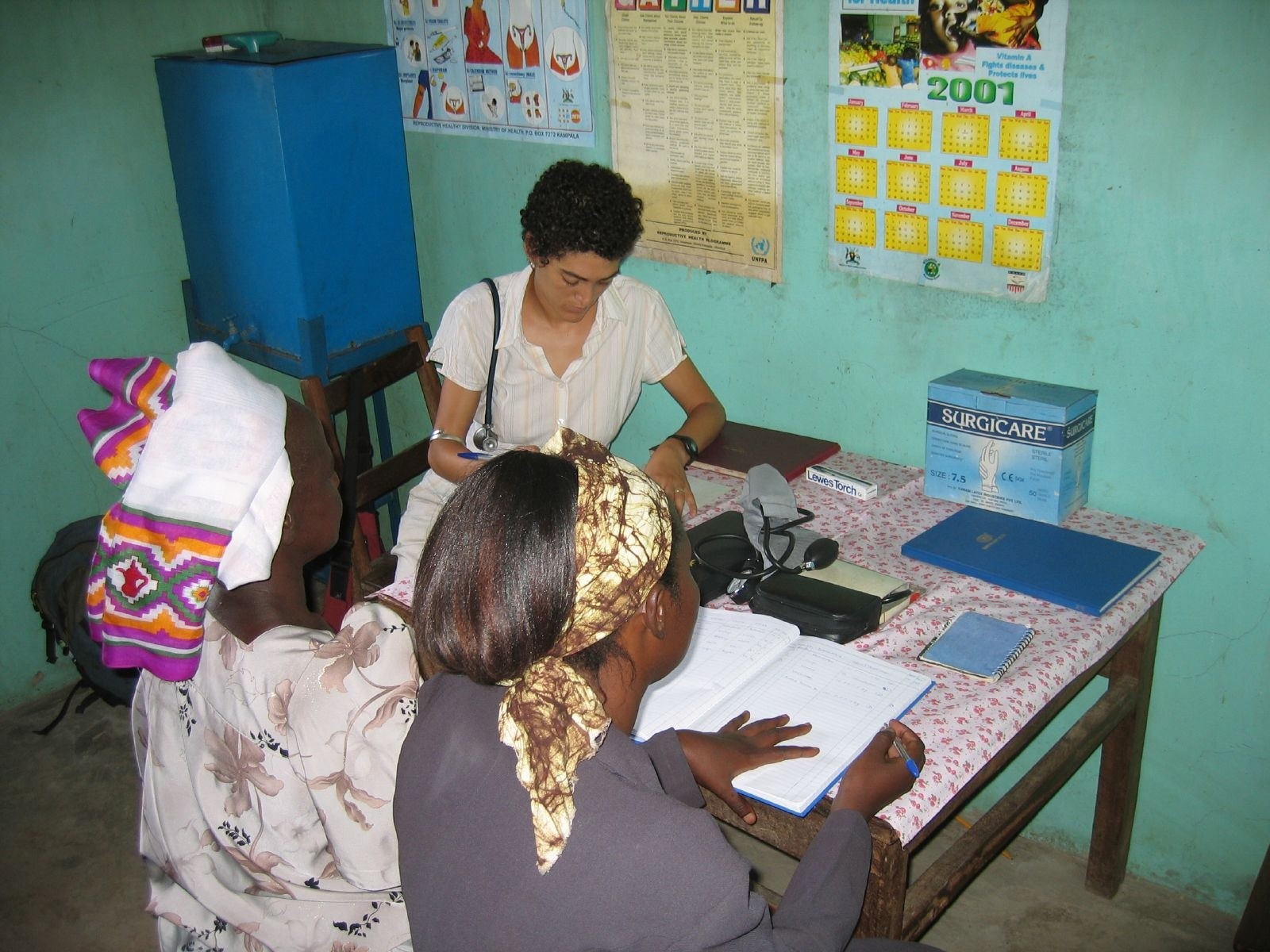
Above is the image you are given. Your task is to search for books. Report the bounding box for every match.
[902,506,1161,617]
[632,606,936,822]
[917,610,1037,682]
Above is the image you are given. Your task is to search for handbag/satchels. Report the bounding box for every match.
[749,570,882,643]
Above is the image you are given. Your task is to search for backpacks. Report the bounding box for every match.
[31,514,141,738]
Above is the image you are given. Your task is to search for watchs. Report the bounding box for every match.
[666,434,698,470]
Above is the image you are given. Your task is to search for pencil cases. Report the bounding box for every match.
[747,572,881,645]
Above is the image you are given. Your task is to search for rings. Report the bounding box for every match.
[674,489,685,494]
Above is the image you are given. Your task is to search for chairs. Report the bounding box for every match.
[296,326,444,609]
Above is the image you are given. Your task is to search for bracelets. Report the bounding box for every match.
[429,429,465,447]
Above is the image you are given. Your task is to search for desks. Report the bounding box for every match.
[373,448,1206,943]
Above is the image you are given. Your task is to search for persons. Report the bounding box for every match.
[392,159,726,582]
[393,426,946,952]
[76,340,422,952]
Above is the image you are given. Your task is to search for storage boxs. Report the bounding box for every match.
[807,464,877,502]
[927,368,1098,528]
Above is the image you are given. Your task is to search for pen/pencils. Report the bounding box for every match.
[457,453,493,461]
[883,722,919,778]
